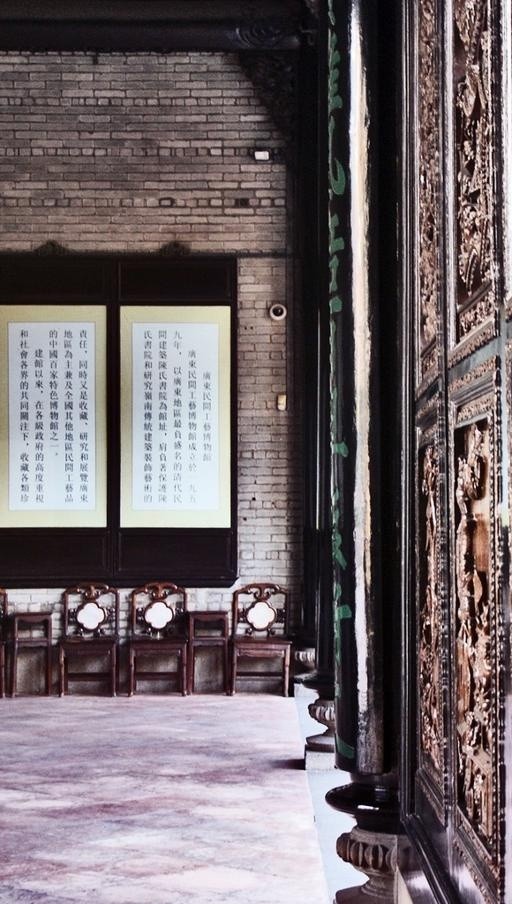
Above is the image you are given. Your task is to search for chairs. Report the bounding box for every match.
[58,581,188,696]
[227,582,293,697]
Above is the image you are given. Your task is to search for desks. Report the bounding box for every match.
[188,610,231,695]
[11,610,53,698]
